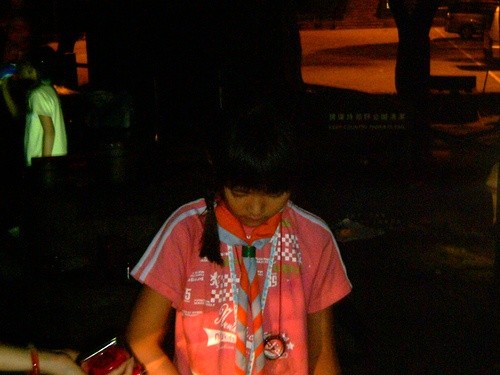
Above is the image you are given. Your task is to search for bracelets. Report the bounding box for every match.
[27,344,38,375]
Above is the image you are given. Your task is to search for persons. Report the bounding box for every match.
[125,106,352,375]
[0,344,91,375]
[0,45,73,259]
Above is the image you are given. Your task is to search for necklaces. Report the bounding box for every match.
[232,216,286,360]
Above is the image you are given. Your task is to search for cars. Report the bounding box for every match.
[375,0,500,70]
